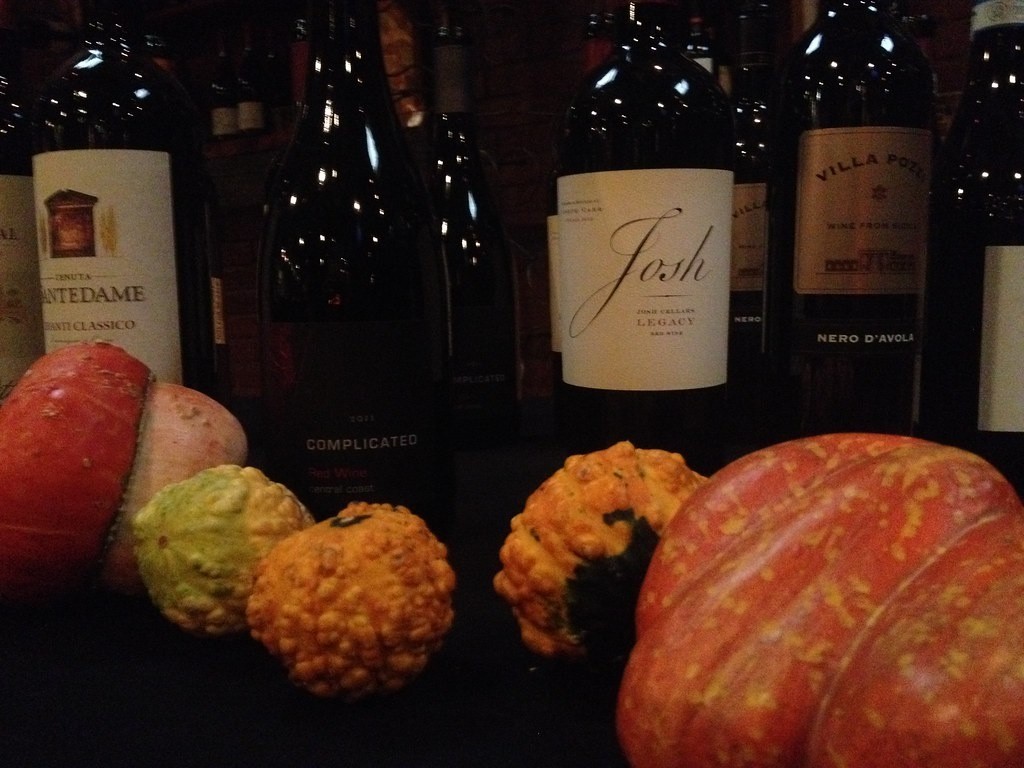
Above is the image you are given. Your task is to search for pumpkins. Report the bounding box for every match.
[614,433,1024,768]
[0,340,154,585]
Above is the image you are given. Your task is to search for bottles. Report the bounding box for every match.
[0,0,1024,523]
